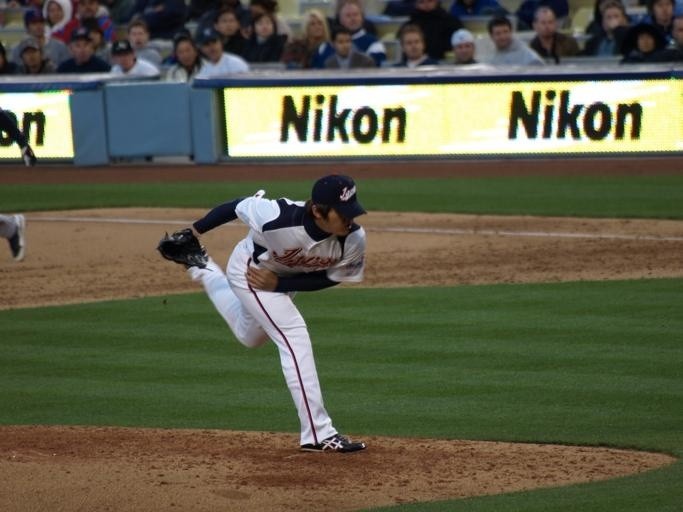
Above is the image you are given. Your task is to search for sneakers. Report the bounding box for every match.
[300,432,365,453]
[8,214,26,260]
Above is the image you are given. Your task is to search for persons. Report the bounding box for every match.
[157,173,367,454]
[0,107,35,263]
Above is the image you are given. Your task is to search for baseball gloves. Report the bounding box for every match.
[157,229,208,268]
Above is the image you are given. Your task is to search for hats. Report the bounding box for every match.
[313,176,368,219]
